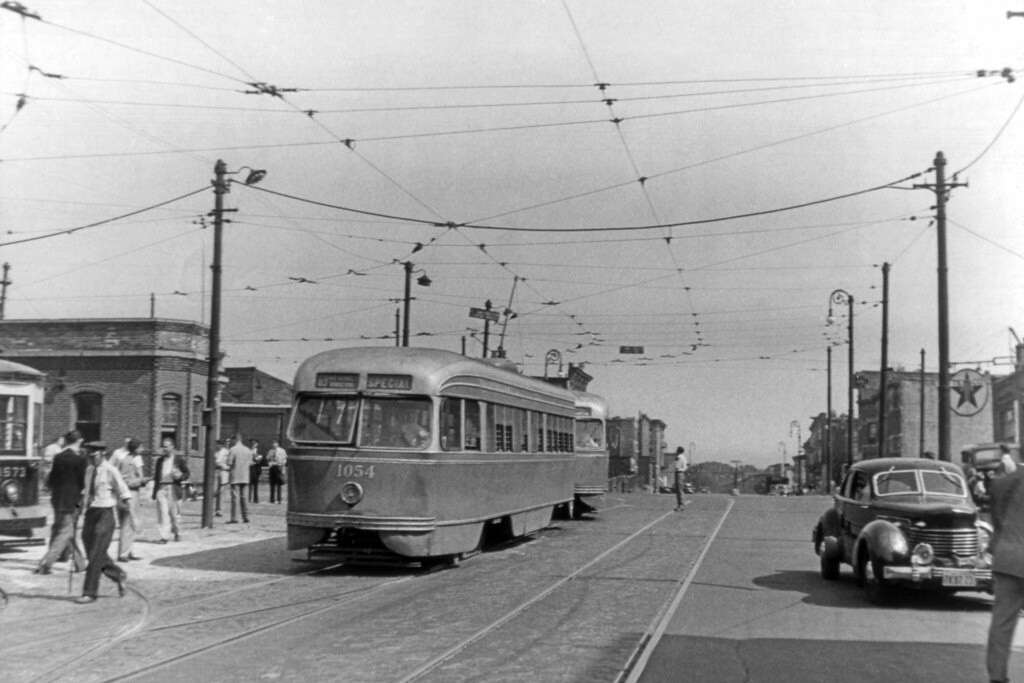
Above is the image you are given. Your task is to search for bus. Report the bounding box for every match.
[0,359,47,532]
[284,347,609,565]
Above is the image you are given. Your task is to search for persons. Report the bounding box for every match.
[215,432,287,523]
[984,444,1024,683]
[382,413,428,447]
[36,431,190,605]
[674,447,687,511]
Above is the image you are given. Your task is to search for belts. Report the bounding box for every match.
[159,482,174,484]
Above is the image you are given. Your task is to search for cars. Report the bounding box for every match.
[960,443,1020,510]
[812,458,995,606]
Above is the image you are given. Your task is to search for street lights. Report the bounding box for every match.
[483,301,513,358]
[201,159,267,525]
[827,289,855,464]
[545,349,565,382]
[404,261,433,347]
[789,420,803,496]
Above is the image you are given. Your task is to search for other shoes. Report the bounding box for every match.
[674,506,684,511]
[118,555,144,563]
[243,519,249,523]
[73,594,98,604]
[35,564,52,574]
[215,512,223,517]
[117,570,128,596]
[159,538,168,544]
[175,532,182,541]
[225,519,239,523]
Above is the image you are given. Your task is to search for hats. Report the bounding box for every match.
[975,471,985,479]
[82,440,107,457]
[249,439,260,443]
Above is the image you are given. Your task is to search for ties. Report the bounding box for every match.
[89,465,98,497]
